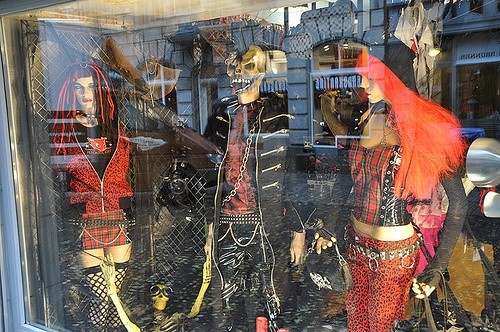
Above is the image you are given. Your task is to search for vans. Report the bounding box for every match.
[58,90,227,208]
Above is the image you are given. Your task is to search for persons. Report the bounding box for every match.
[49,28,471,332]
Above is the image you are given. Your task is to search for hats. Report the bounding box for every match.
[369,35,419,93]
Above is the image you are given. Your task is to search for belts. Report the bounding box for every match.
[347,233,424,261]
[65,219,135,228]
[220,214,261,224]
[351,213,414,241]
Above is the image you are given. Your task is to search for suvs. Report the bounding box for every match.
[202,85,368,210]
